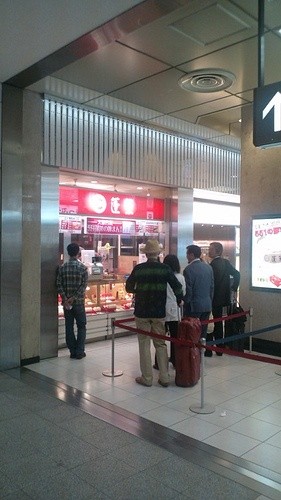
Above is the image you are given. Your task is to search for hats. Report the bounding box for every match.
[140,238,165,254]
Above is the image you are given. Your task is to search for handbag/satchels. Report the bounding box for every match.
[232,298,247,322]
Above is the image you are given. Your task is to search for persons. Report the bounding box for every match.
[56,243,88,359]
[126,240,184,387]
[204,242,240,357]
[183,245,214,337]
[153,254,186,370]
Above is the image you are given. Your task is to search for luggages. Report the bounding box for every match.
[224,289,245,353]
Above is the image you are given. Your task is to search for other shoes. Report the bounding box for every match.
[135,376,152,387]
[152,365,158,369]
[158,378,168,387]
[70,352,86,359]
